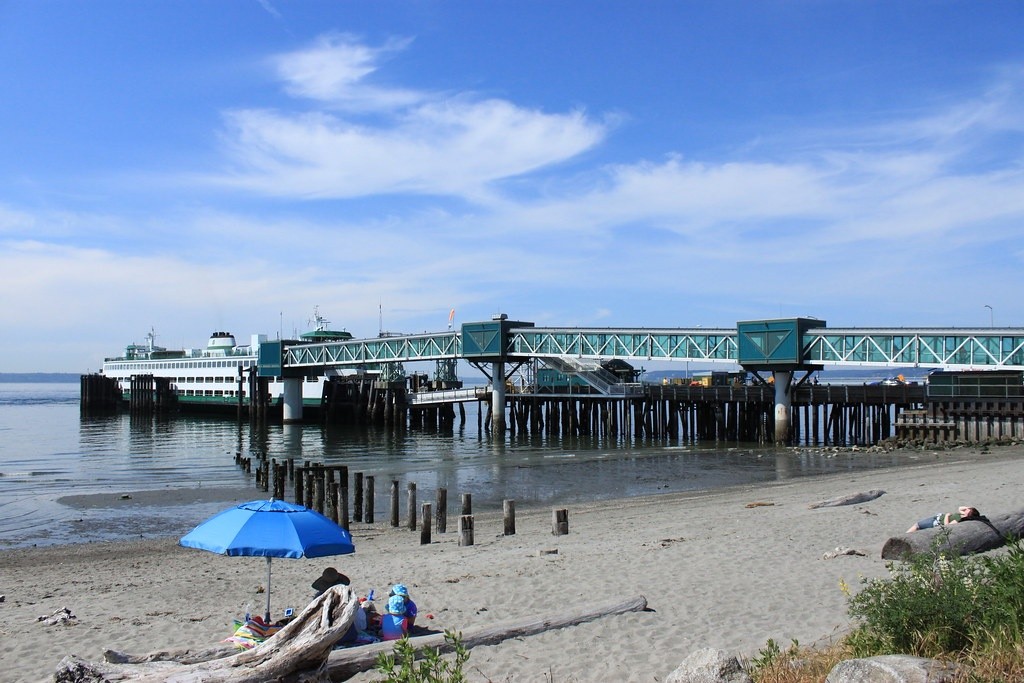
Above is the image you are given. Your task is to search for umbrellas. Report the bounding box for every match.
[176,497,356,626]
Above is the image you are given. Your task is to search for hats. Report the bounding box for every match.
[385,596,406,614]
[312,567,350,590]
[387,585,408,599]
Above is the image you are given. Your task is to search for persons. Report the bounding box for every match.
[807,377,810,383]
[276,567,417,646]
[814,376,818,386]
[753,378,758,386]
[663,378,668,385]
[906,506,980,533]
